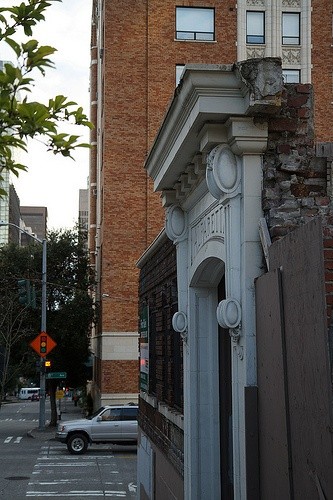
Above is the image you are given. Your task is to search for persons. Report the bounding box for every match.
[30,388,94,417]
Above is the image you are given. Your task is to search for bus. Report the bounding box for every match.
[19,388,41,401]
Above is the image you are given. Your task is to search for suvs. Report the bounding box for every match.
[56,403,139,456]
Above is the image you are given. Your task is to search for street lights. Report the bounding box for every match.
[0,222,48,430]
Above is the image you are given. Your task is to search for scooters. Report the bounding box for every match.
[31,395,39,401]
